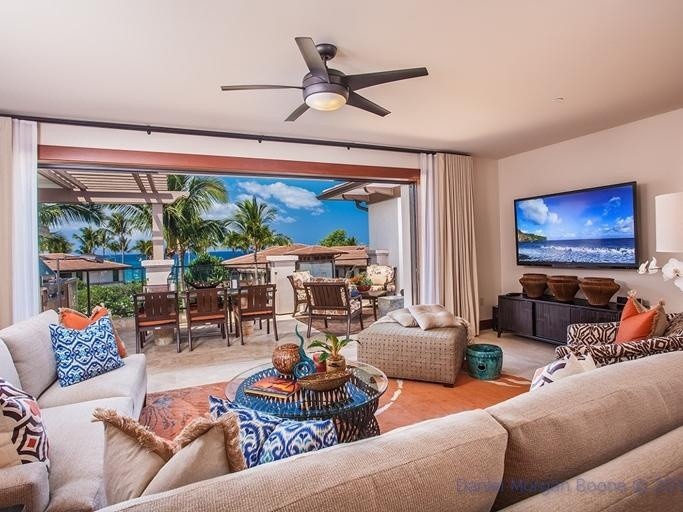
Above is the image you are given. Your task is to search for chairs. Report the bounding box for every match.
[132,264,397,353]
[555,313,683,368]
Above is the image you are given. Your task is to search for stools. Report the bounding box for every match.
[356,315,467,388]
[466,344,503,380]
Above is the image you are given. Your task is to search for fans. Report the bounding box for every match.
[221,37,429,121]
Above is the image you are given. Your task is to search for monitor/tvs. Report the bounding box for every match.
[512,180,638,270]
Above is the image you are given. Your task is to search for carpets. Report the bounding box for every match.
[138,360,532,440]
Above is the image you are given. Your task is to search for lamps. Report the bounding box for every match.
[303,83,350,112]
[655,192,683,253]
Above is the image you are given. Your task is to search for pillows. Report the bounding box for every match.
[389,307,419,327]
[0,378,52,480]
[91,406,247,506]
[614,290,667,344]
[58,303,128,358]
[408,304,462,331]
[530,352,597,390]
[208,395,339,469]
[48,315,125,388]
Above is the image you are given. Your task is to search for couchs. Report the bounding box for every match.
[1,310,146,512]
[95,350,683,512]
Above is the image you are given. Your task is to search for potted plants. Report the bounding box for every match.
[306,331,362,373]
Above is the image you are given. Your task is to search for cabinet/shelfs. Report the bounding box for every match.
[498,293,622,345]
